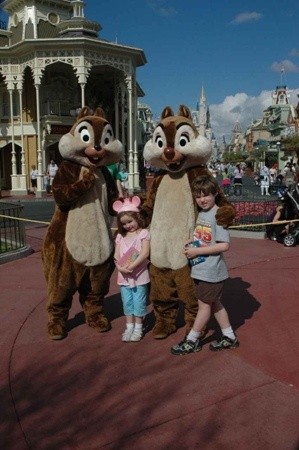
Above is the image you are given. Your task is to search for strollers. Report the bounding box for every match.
[276,178,299,247]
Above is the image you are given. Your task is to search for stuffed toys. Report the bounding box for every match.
[143,105,236,342]
[41,106,124,339]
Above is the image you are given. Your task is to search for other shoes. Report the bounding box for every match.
[267,193,269,195]
[261,193,263,195]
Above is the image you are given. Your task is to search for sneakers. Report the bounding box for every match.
[122,329,133,341]
[210,336,239,351]
[134,330,142,341]
[171,336,201,355]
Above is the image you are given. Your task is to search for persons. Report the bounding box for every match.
[171,175,238,354]
[30,164,38,195]
[47,160,58,193]
[207,160,299,197]
[113,195,151,342]
